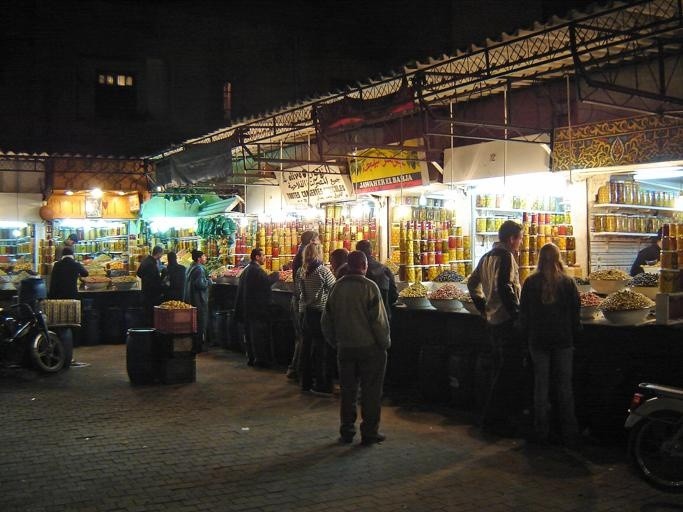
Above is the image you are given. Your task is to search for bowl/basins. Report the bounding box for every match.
[212,275,235,284]
[80,276,139,290]
[397,281,480,313]
[579,280,659,326]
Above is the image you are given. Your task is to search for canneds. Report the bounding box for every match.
[235,219,376,271]
[594,181,683,293]
[399,204,472,283]
[476,193,581,286]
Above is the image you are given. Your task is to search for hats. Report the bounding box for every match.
[192,249,205,260]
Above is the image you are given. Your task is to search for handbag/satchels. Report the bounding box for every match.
[300,310,321,331]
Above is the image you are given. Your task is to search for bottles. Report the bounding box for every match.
[389,205,474,280]
[592,185,672,232]
[319,206,381,263]
[510,211,583,283]
[475,195,503,232]
[59,222,127,260]
[235,219,309,271]
[660,221,683,291]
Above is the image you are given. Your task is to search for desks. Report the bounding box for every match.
[0,285,683,445]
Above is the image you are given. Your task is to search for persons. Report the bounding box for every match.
[517,242,583,449]
[243,249,281,369]
[136,245,217,352]
[232,269,253,367]
[46,246,88,299]
[286,230,399,444]
[56,233,78,261]
[467,221,530,431]
[630,227,663,277]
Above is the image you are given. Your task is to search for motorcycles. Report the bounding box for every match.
[625,381,683,492]
[0,266,66,374]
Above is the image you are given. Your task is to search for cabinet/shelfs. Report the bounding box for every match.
[592,204,683,237]
[476,207,565,235]
[0,226,128,257]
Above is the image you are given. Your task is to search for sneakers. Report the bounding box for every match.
[362,432,385,446]
[310,384,334,396]
[285,367,297,378]
